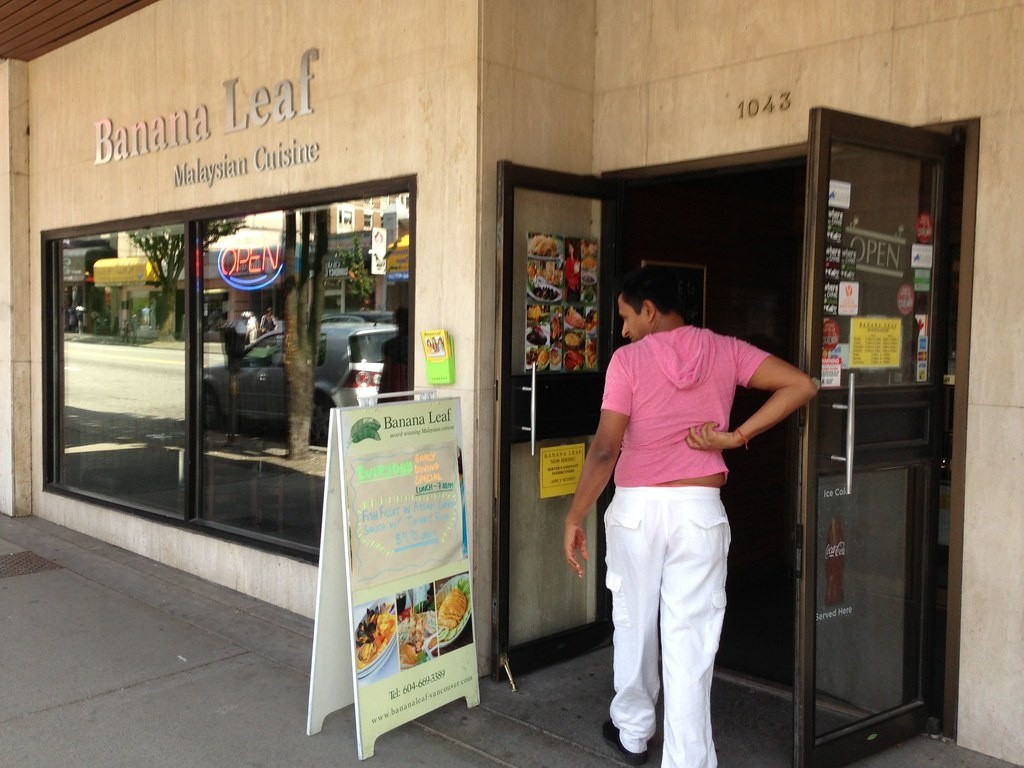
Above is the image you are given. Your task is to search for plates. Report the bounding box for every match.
[527,236,599,373]
[356,597,439,683]
[434,573,473,646]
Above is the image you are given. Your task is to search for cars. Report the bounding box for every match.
[202,322,400,450]
[320,307,398,323]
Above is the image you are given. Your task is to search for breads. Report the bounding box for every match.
[398,644,418,664]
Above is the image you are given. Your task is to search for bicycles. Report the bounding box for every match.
[121,314,137,344]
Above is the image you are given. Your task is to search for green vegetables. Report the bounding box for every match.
[448,578,470,594]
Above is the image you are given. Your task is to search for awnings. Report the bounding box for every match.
[63,247,107,281]
[387,234,409,280]
[94,257,160,283]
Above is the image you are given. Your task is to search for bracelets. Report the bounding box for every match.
[736,430,749,449]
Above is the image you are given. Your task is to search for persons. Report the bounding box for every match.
[142,298,158,329]
[564,264,818,768]
[221,307,275,360]
[377,308,408,404]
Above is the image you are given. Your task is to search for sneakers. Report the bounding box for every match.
[603,718,647,765]
